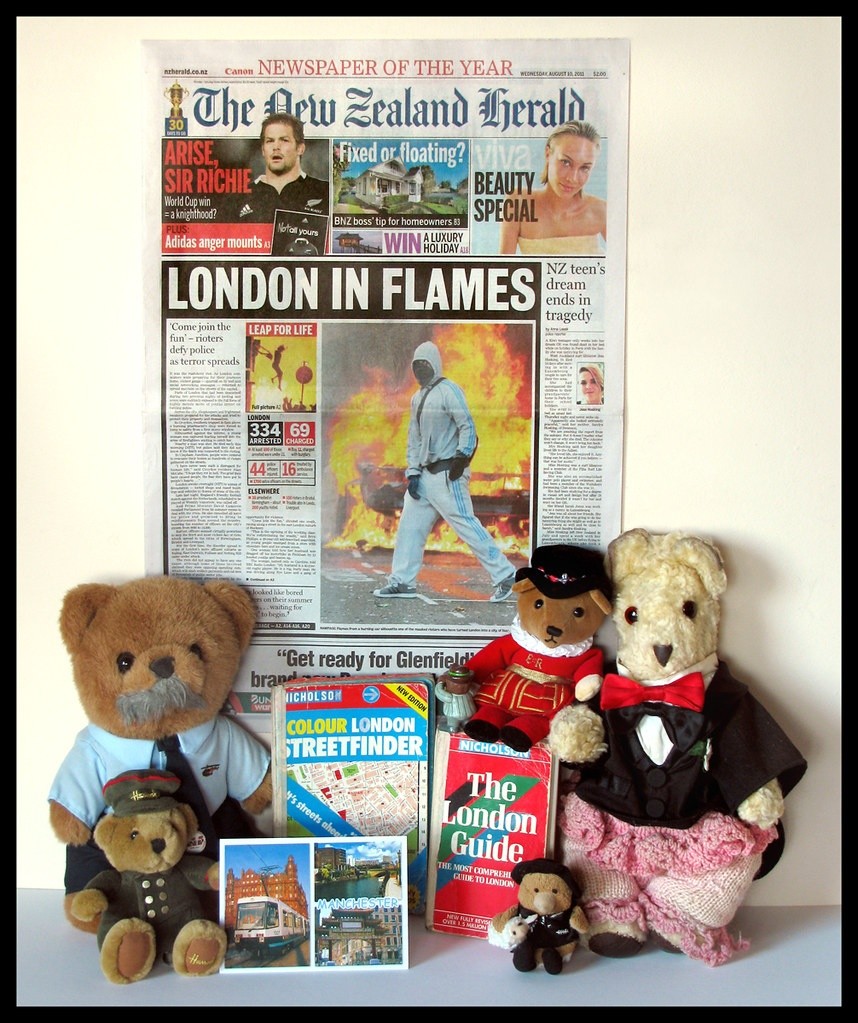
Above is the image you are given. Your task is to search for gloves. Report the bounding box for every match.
[408,475,421,499]
[448,457,465,482]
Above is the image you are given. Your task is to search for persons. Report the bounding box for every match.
[369,341,520,603]
[251,339,270,372]
[578,365,604,405]
[270,345,285,391]
[216,114,330,223]
[498,119,607,254]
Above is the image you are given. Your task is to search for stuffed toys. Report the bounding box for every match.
[486,857,590,974]
[544,530,808,965]
[68,769,228,985]
[465,543,618,753]
[46,575,274,933]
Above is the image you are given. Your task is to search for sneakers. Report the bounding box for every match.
[490,570,517,602]
[374,582,417,598]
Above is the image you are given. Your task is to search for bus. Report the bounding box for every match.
[232,865,310,959]
